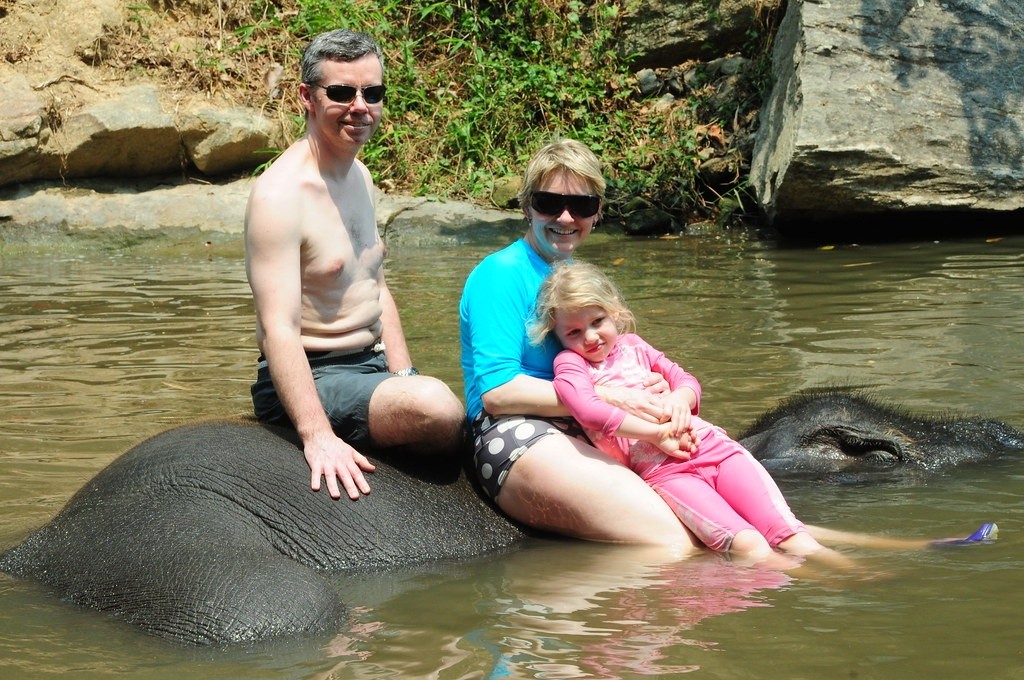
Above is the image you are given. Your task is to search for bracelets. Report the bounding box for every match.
[394,367,419,376]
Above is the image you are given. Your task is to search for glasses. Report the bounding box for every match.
[529,191,600,217]
[313,82,386,104]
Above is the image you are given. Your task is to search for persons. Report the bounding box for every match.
[458,138,1000,549]
[243,29,466,501]
[524,258,825,558]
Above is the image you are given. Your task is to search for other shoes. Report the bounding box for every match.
[932,521,1000,553]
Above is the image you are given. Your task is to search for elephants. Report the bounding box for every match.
[0,382,1023,650]
